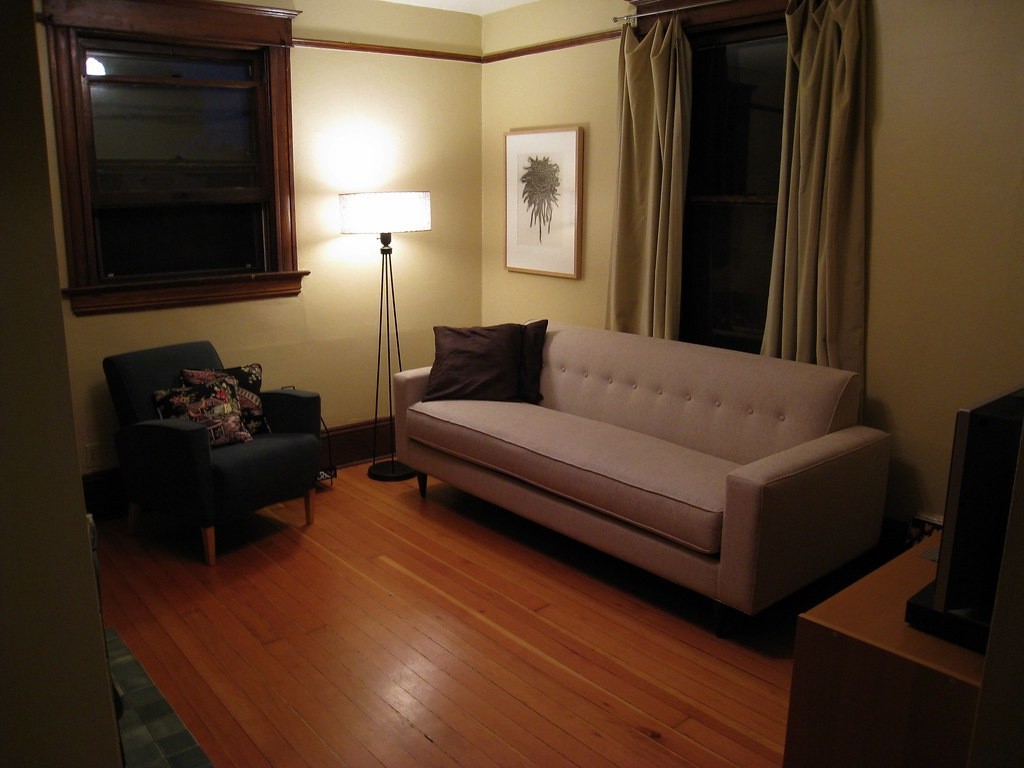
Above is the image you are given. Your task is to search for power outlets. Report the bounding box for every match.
[85,442,104,467]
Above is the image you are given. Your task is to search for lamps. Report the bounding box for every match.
[338,190,431,482]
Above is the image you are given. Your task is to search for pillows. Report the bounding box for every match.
[519,320,548,405]
[179,362,272,436]
[153,375,253,448]
[421,322,528,403]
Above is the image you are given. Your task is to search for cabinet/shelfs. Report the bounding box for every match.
[782,530,985,768]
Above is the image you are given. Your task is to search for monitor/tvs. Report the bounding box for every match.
[932,386,1024,614]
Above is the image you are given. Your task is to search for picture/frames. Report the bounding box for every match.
[503,127,584,280]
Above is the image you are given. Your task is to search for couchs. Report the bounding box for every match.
[393,317,891,639]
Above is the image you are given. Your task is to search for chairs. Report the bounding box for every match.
[102,341,322,568]
